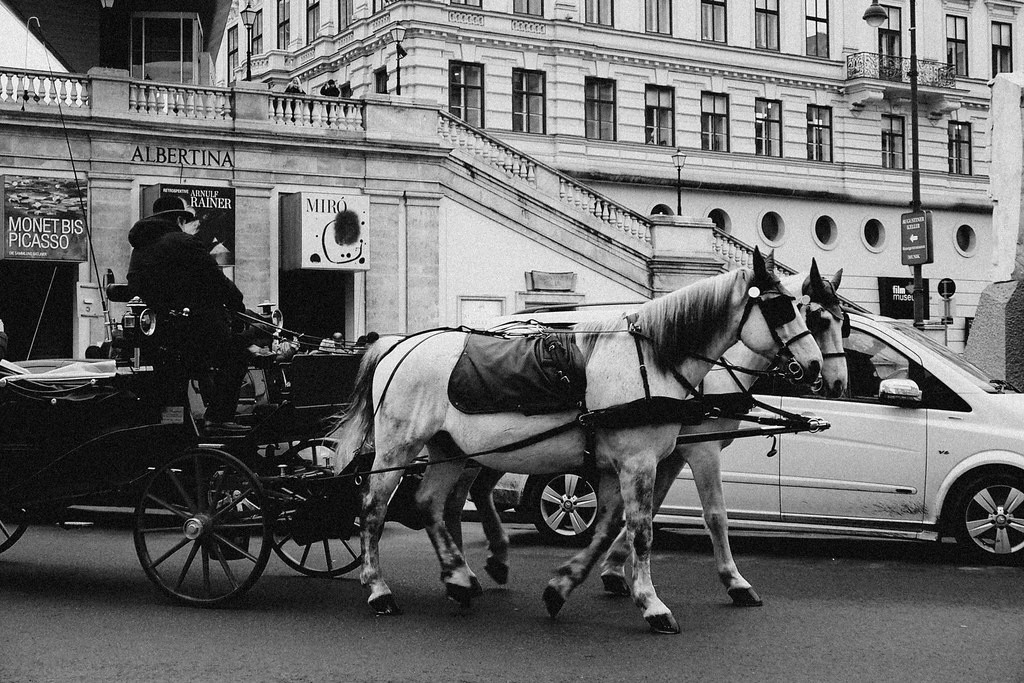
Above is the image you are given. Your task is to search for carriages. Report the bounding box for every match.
[0,245,852,634]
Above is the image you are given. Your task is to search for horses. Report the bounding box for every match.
[320,243,851,636]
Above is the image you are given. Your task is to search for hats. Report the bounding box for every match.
[319,338,336,353]
[145,197,194,224]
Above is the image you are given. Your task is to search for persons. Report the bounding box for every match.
[284,76,306,122]
[128,193,279,434]
[271,331,380,387]
[321,79,340,125]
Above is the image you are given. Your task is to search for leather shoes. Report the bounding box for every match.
[205,422,253,433]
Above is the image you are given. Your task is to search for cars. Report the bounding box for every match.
[459,285,1023,566]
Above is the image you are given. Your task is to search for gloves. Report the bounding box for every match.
[226,300,245,317]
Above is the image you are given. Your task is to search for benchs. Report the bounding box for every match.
[254,352,361,437]
